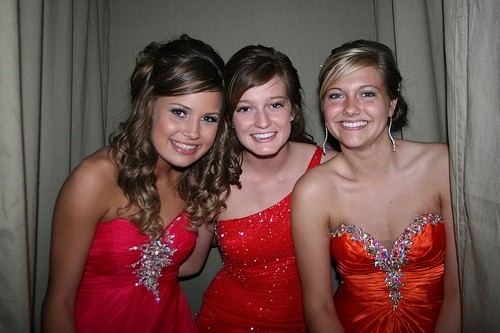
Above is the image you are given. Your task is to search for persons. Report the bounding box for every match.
[40,33,243,333]
[291,39,463,333]
[178,44,341,333]
